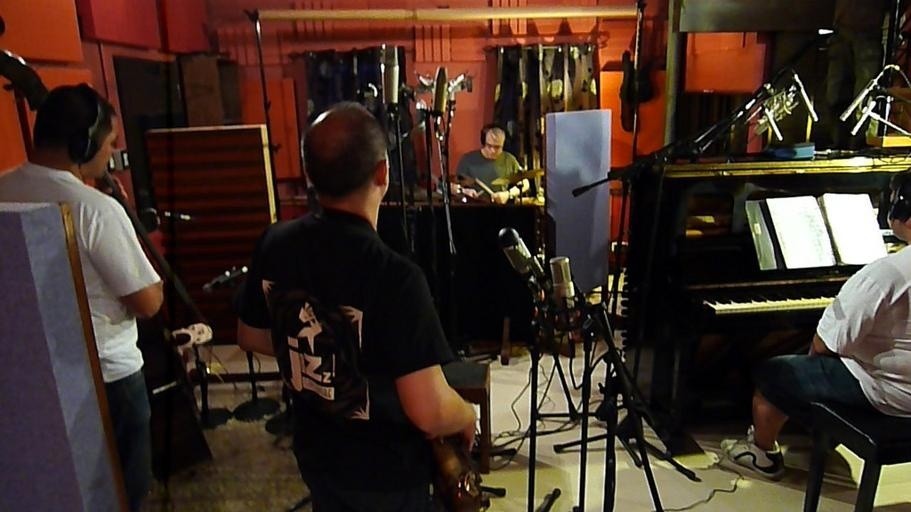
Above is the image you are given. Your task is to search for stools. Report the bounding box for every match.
[446,362,495,475]
[800,402,911,512]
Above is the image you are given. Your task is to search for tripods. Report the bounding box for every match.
[527,0,702,512]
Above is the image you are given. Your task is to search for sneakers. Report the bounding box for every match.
[720,439,785,481]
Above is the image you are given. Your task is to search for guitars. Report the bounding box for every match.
[425,431,483,512]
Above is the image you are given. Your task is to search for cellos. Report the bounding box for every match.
[0,50,215,480]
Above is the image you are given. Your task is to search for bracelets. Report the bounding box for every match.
[507,190,513,200]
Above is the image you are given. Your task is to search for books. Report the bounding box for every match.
[765,193,888,269]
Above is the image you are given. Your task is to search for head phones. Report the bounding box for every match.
[68,97,105,164]
[480,121,511,150]
[888,179,911,224]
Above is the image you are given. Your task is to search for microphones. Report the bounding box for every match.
[762,107,784,142]
[790,68,819,123]
[498,226,535,275]
[839,73,884,122]
[202,266,248,294]
[850,99,877,136]
[379,43,473,114]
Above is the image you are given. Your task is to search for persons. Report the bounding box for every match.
[0,83,164,511]
[721,168,911,482]
[234,100,476,512]
[456,127,530,205]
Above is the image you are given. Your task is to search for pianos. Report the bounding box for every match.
[617,151,911,457]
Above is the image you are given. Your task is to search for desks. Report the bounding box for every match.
[380,201,546,365]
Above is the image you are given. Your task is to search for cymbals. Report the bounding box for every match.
[492,169,545,185]
